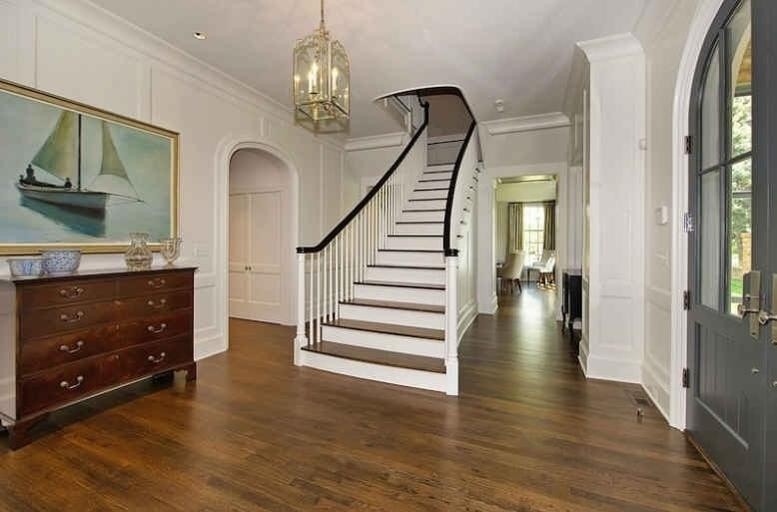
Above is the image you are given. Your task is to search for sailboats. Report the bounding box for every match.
[15,108,147,219]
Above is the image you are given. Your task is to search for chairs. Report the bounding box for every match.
[496,248,555,295]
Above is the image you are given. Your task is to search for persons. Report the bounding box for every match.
[26,164,34,180]
[63,177,72,188]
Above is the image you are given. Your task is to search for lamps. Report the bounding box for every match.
[292,1,351,135]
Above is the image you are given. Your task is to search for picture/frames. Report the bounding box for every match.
[0,78,180,257]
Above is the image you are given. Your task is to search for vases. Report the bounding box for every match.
[123,231,153,271]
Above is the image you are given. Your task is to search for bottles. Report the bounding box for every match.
[123,231,155,269]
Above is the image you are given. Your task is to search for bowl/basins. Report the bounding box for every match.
[5,247,84,279]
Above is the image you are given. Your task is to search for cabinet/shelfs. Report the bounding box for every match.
[1,265,200,451]
